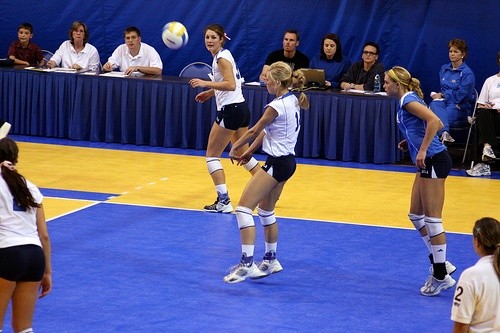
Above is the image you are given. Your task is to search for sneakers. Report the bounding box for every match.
[257,259,284,273]
[482,146,496,161]
[466,163,491,176]
[419,274,457,296]
[204,196,234,212]
[223,261,261,284]
[429,261,456,276]
[441,133,456,146]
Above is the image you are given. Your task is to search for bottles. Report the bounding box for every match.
[374,73,382,93]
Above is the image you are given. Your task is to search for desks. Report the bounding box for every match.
[0,68,419,172]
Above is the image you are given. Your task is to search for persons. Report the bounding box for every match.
[465,51,500,177]
[429,39,475,148]
[451,217,500,333]
[8,23,45,67]
[0,138,53,333]
[102,27,163,76]
[46,21,100,72]
[340,41,385,92]
[309,33,352,89]
[224,62,309,282]
[188,24,261,213]
[382,66,457,295]
[260,29,310,82]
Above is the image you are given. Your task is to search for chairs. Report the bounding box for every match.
[179,63,214,79]
[447,87,479,165]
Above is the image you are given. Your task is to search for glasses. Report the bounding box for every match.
[363,51,377,56]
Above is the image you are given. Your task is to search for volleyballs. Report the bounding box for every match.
[161,21,188,50]
